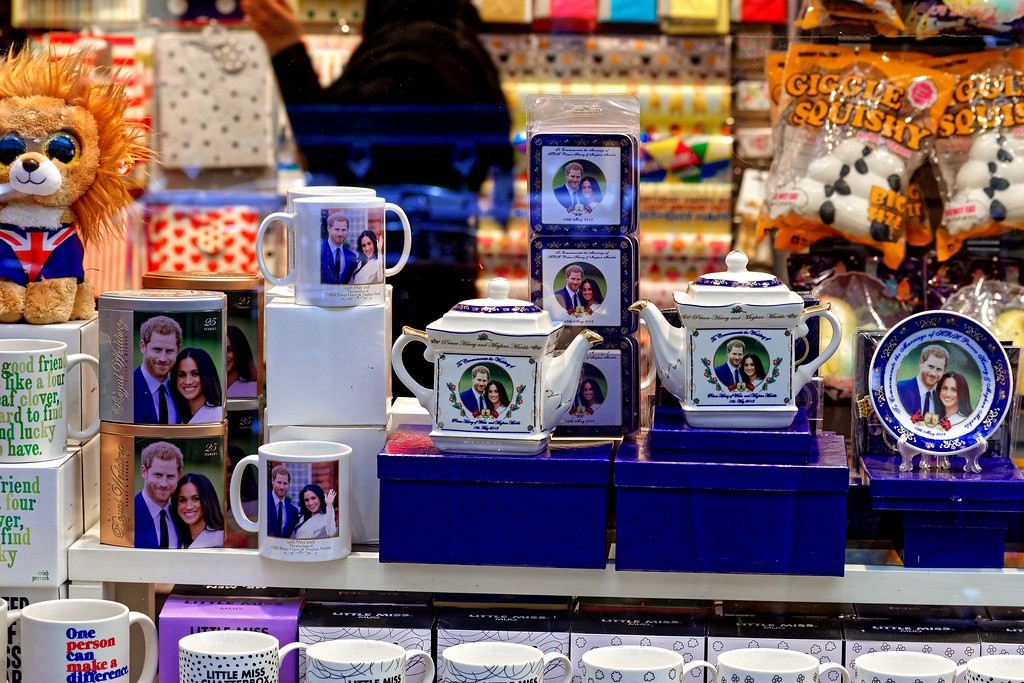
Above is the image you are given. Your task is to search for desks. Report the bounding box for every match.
[65,522,1024,683]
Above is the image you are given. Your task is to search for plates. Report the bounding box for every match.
[868,310,1015,456]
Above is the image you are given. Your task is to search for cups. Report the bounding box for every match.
[256,185,411,309]
[0,596,22,683]
[306,639,435,683]
[20,599,159,683]
[230,439,354,562]
[967,655,1024,683]
[582,645,718,683]
[178,629,309,683]
[719,648,851,683]
[0,339,99,465]
[855,650,966,683]
[442,641,573,683]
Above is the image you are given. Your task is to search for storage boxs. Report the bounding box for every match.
[613,433,850,576]
[65,434,100,533]
[840,618,983,683]
[570,609,708,683]
[376,423,616,569]
[295,601,435,683]
[856,455,1024,514]
[434,605,571,683]
[0,447,86,591]
[892,510,1009,569]
[157,594,305,683]
[263,284,393,546]
[0,312,99,447]
[970,620,1024,683]
[0,581,68,683]
[706,612,843,683]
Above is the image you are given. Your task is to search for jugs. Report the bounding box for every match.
[628,249,841,429]
[390,276,605,456]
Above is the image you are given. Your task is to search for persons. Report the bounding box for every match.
[937,371,973,426]
[267,465,338,539]
[740,354,767,389]
[237,0,515,400]
[132,315,222,425]
[557,367,606,415]
[224,324,258,400]
[225,443,258,517]
[897,345,945,422]
[554,162,603,215]
[555,266,604,314]
[460,366,511,417]
[714,340,746,388]
[134,440,223,549]
[321,213,384,285]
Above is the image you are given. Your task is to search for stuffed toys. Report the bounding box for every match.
[0,35,161,325]
